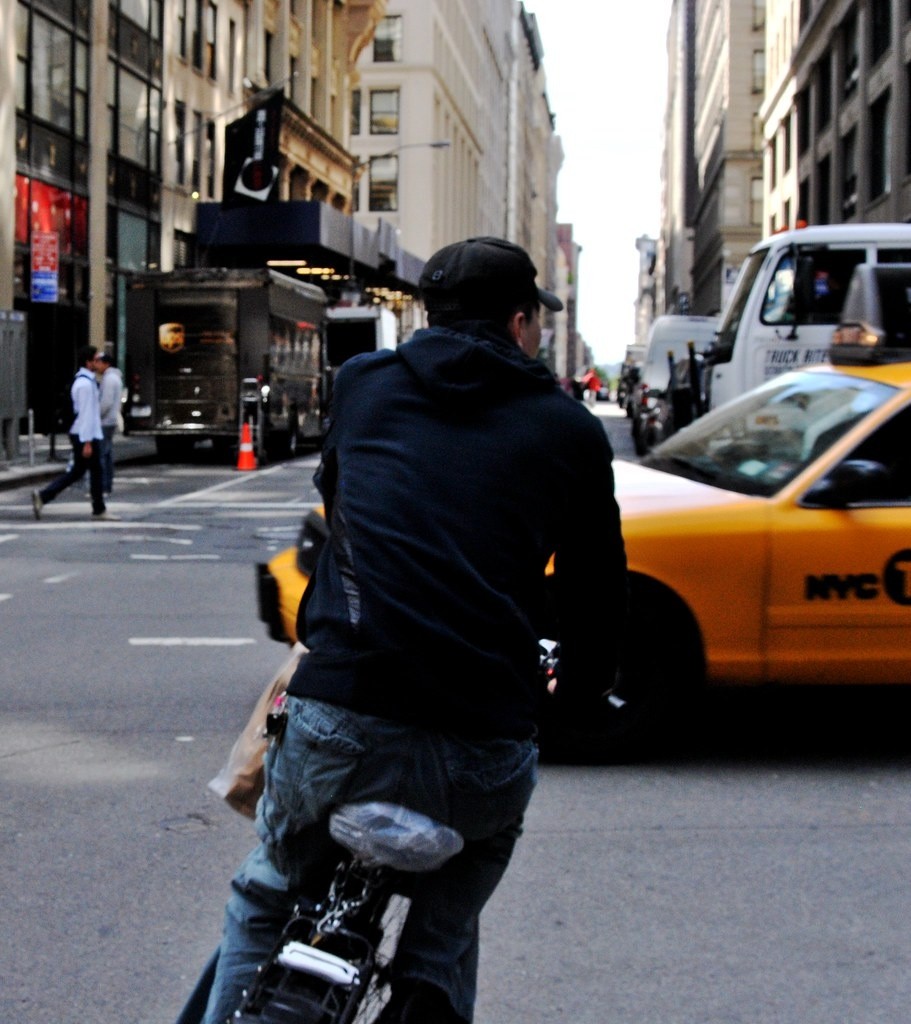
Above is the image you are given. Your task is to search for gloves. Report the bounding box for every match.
[553,665,620,716]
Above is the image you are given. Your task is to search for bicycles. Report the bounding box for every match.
[176,510,623,1024]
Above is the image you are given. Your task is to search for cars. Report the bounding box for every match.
[257,363,911,764]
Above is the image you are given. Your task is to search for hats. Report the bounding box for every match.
[419,238,564,313]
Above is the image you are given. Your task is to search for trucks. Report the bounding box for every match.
[120,266,400,465]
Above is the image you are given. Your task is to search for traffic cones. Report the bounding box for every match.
[236,423,259,471]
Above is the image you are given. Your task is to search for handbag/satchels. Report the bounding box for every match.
[206,642,310,820]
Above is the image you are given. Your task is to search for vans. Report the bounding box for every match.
[618,223,911,457]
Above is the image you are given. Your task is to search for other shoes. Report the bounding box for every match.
[92,512,121,520]
[374,978,468,1024]
[32,490,44,520]
[84,492,108,497]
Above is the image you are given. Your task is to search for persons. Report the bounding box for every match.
[176,237,629,1024]
[84,352,123,498]
[31,346,121,521]
